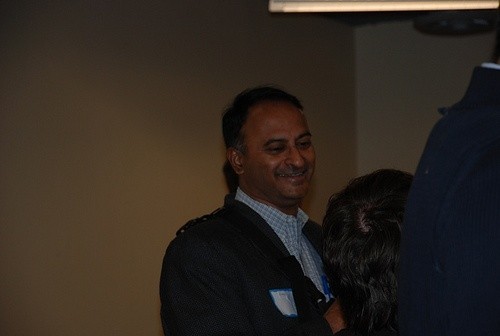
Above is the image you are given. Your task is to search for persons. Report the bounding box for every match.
[158,82,350,336]
[397,54,500,336]
[321,167,416,336]
